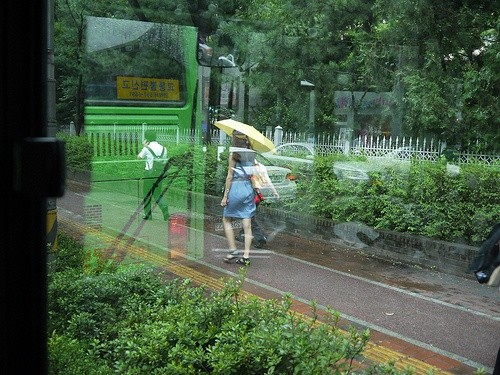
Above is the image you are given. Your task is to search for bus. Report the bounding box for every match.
[81,13,206,280]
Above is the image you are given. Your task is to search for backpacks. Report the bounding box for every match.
[145,146,164,177]
[466,223,500,285]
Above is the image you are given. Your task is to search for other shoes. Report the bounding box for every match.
[235,237,244,243]
[144,216,152,220]
[164,214,170,221]
[254,234,268,248]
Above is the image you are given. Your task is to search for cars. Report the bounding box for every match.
[259,142,374,184]
[377,146,462,182]
[203,146,297,206]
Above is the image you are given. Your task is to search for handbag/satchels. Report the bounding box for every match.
[254,193,263,204]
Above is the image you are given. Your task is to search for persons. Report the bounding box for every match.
[221,129,257,265]
[138,129,170,221]
[235,156,280,248]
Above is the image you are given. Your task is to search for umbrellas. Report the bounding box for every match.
[214,119,277,155]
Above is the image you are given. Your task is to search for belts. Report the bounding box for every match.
[232,177,250,180]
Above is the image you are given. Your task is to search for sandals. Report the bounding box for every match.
[235,257,250,265]
[223,250,239,262]
